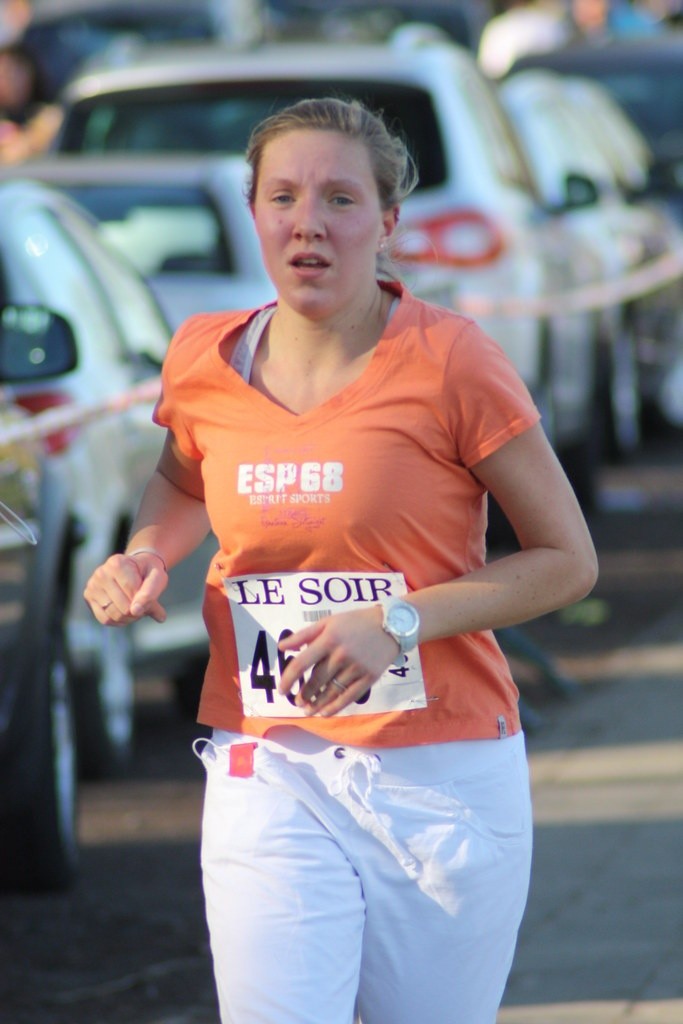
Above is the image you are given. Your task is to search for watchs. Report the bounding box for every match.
[376,597,420,654]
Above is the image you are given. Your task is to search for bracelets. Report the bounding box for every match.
[127,547,168,572]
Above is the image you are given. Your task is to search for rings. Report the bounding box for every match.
[102,601,113,610]
[332,678,346,690]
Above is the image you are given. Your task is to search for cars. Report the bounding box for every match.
[18,1,681,522]
[2,167,214,902]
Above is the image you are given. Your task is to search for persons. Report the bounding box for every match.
[0,43,65,164]
[84,97,599,1024]
